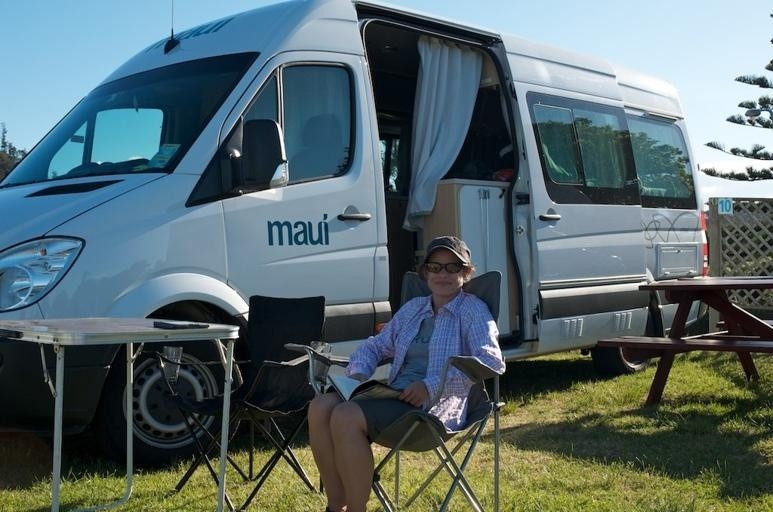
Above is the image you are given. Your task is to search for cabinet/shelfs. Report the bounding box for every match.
[417,176,520,349]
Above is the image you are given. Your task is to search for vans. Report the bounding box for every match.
[0,0,708,471]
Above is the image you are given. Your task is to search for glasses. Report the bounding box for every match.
[425,261,463,275]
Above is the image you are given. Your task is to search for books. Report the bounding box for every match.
[327,373,403,403]
[153,319,210,329]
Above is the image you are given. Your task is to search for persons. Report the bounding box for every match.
[306,235,506,512]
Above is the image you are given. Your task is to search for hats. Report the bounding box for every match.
[421,236,471,267]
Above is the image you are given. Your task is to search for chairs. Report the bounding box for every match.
[286,271,503,511]
[155,296,332,508]
[285,114,345,180]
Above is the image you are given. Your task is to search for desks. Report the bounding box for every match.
[634,275,773,410]
[0,317,241,512]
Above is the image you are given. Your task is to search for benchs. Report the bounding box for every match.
[594,318,773,408]
